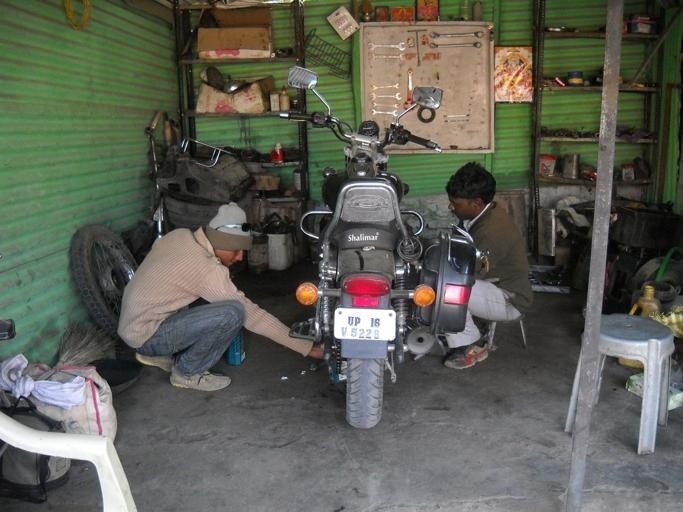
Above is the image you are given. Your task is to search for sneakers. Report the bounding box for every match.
[168,370,232,393]
[134,350,174,372]
[442,340,490,370]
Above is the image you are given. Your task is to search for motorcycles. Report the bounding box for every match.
[274,63,482,431]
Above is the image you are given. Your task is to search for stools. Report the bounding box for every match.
[563,312,675,456]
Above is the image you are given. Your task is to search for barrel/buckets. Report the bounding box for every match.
[539,155,556,176]
[248,235,269,276]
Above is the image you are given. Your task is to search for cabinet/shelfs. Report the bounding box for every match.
[172,1,310,273]
[530,0,666,261]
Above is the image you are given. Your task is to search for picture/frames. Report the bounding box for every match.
[492,44,536,106]
[375,0,440,22]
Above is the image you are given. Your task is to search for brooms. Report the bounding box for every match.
[35,321,118,381]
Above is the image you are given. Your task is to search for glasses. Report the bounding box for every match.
[213,221,252,233]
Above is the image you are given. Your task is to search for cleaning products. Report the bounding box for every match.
[228,328,246,365]
[619,285,662,367]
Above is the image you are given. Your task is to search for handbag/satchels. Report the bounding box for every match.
[0,395,71,496]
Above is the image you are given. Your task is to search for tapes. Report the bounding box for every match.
[416,106,436,124]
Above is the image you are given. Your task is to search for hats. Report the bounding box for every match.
[203,200,252,252]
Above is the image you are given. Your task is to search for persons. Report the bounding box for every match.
[112,201,325,393]
[415,161,532,372]
